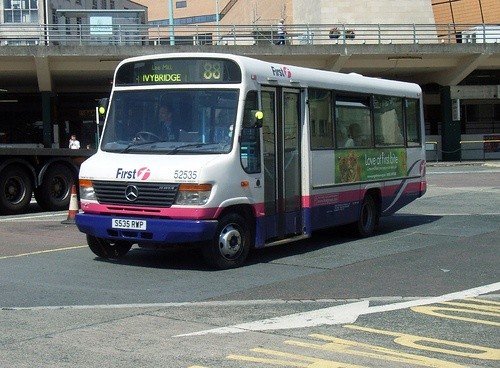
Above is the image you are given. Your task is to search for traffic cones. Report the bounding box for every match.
[62,185,83,225]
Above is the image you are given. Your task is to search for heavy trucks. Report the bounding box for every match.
[0,120,97,211]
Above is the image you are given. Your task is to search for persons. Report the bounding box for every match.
[156,105,181,141]
[274,18,286,45]
[344,123,363,147]
[69,135,81,149]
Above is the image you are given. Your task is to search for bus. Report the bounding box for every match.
[77,52,428,268]
